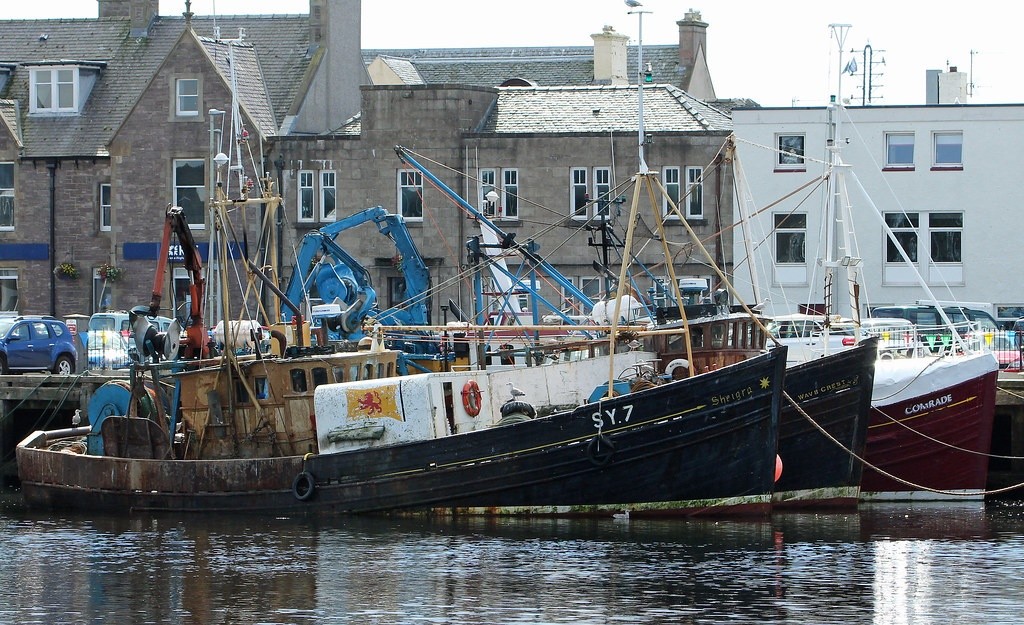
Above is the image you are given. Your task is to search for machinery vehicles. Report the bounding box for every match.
[267,203,471,377]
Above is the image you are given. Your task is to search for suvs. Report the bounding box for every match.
[0,315,79,376]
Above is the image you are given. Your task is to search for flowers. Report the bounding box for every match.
[61,261,80,280]
[95,263,123,283]
[393,255,403,272]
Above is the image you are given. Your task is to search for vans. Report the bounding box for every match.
[87,310,184,362]
[870,304,1014,370]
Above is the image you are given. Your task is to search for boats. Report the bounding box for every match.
[0,0,1023,519]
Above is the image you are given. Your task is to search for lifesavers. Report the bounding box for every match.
[463,379,482,417]
[292,472,315,501]
[587,435,616,466]
[664,358,698,384]
